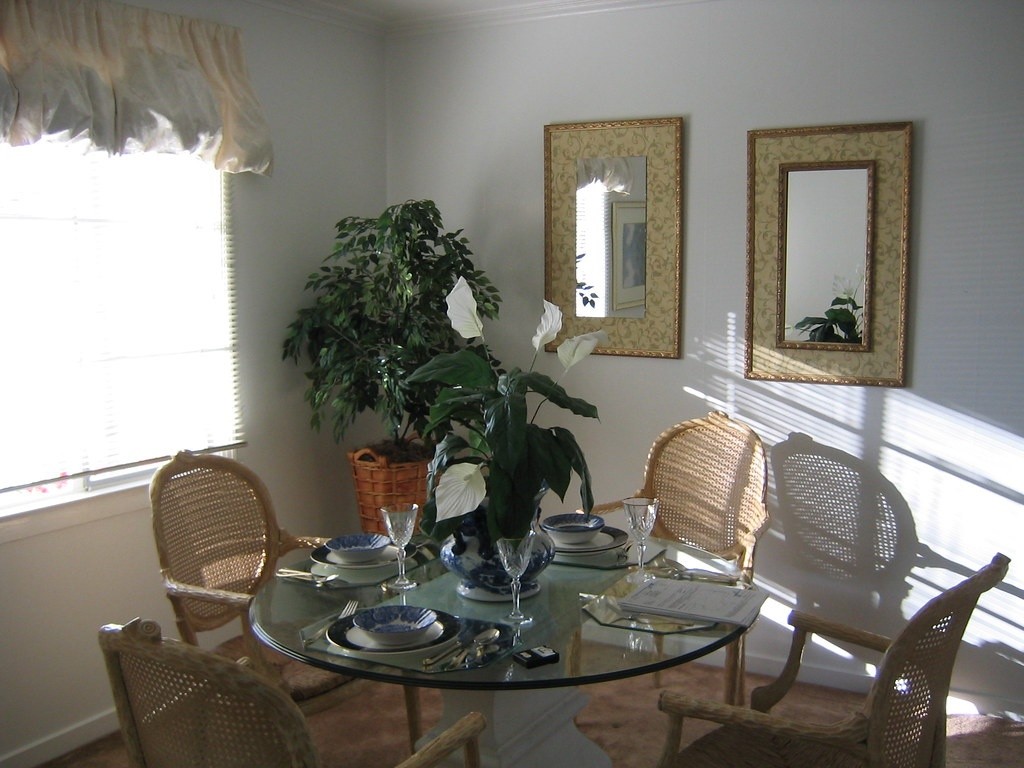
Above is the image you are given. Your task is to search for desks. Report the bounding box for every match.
[249,534,761,768]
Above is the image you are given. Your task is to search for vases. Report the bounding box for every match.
[440,475,556,602]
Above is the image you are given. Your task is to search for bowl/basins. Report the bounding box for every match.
[542,514,605,544]
[353,605,437,645]
[352,605,437,645]
[325,535,390,563]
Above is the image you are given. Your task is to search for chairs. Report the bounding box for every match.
[568,407,772,706]
[148,448,425,768]
[655,552,1012,768]
[98,617,489,768]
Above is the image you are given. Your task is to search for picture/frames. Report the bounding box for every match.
[611,201,646,311]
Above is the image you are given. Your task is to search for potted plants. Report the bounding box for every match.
[280,199,508,535]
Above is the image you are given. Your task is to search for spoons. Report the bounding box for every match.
[277,572,338,584]
[443,629,501,671]
[617,541,634,563]
[663,557,739,581]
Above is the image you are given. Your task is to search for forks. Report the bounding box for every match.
[301,600,359,647]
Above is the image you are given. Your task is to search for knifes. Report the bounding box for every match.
[423,622,484,666]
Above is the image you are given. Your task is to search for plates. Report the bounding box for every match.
[326,609,462,654]
[554,526,628,555]
[309,545,417,569]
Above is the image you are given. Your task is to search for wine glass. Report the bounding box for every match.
[496,538,534,624]
[379,503,419,589]
[621,496,659,583]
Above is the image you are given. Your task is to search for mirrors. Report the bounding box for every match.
[744,120,913,388]
[542,116,685,360]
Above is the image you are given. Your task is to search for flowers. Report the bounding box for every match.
[406,275,611,550]
[785,261,865,344]
